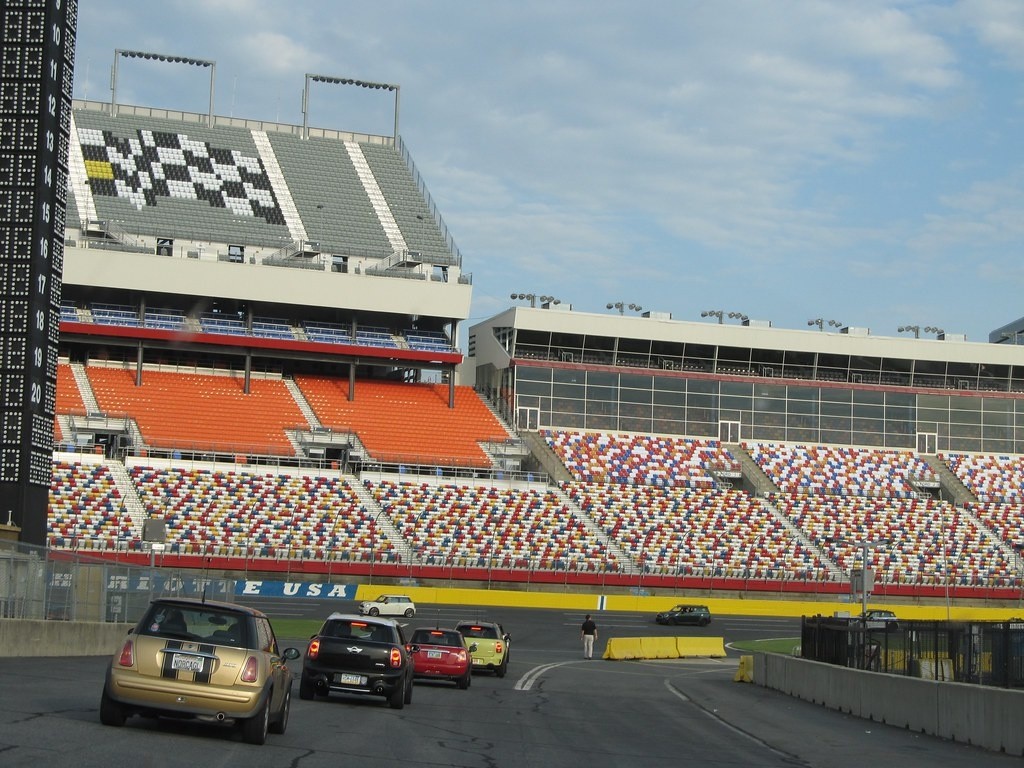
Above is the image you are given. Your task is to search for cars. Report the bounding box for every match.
[357,594,416,618]
[446,619,511,678]
[851,609,900,634]
[655,605,711,627]
[100,597,302,745]
[408,627,477,689]
[300,610,420,709]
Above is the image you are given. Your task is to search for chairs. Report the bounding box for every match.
[338,623,351,636]
[213,624,240,639]
[371,630,383,642]
[162,611,188,632]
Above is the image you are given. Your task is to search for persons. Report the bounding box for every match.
[580,615,598,660]
[165,610,183,623]
[683,609,687,612]
